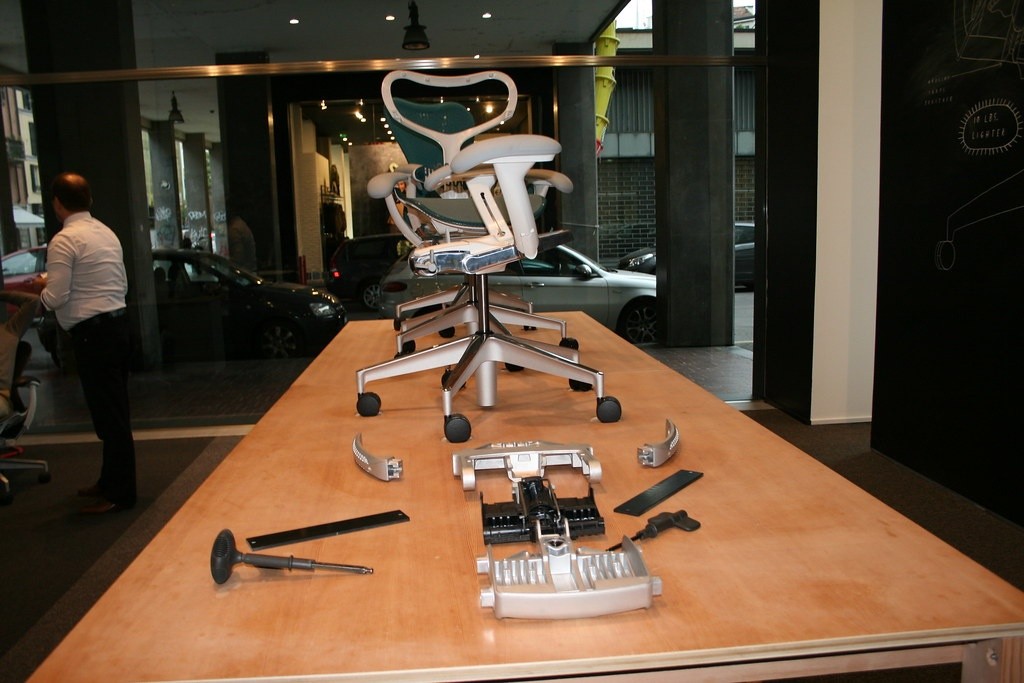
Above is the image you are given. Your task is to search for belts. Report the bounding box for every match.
[66,308,128,339]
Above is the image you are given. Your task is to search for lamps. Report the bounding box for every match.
[401,0,430,50]
[168,91,184,124]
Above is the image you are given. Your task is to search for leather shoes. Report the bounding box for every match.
[79,483,105,496]
[80,498,122,513]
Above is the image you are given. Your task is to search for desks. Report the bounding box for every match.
[26,311,1024,683]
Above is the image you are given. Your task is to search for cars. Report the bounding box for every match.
[320,230,412,312]
[1,245,49,319]
[376,241,656,344]
[617,220,756,290]
[150,247,350,358]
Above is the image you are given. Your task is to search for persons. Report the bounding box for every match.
[34,174,139,505]
[0,290,41,418]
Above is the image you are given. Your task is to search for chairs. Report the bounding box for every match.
[167,266,176,296]
[154,268,170,297]
[0,341,52,506]
